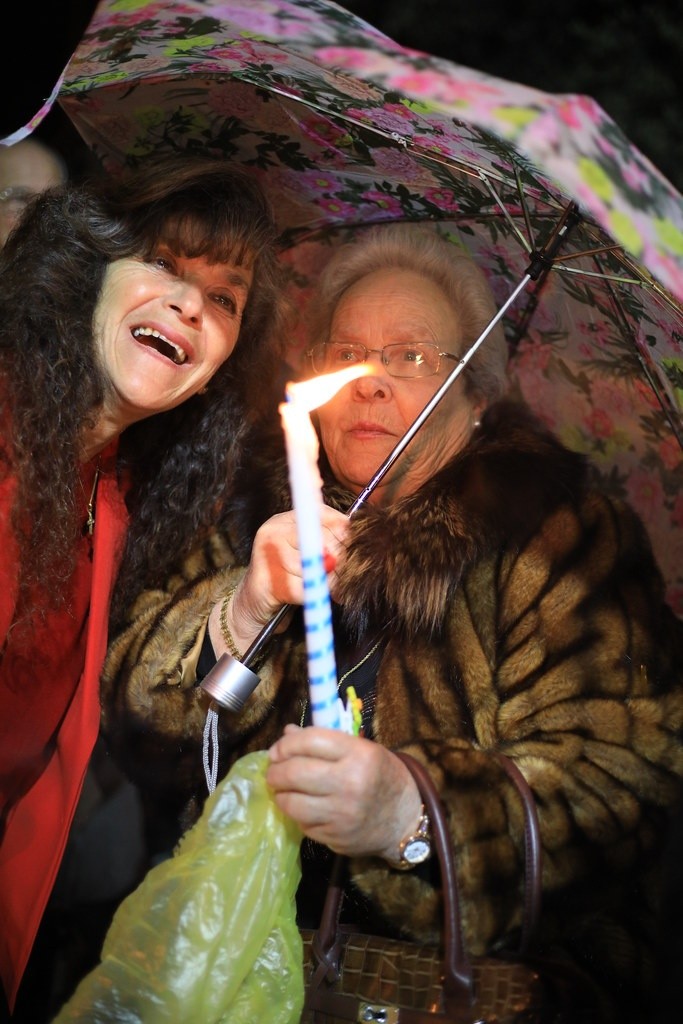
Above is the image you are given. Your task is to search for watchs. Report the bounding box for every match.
[388,802,430,870]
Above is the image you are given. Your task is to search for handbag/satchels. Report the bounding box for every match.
[298,737,547,1024]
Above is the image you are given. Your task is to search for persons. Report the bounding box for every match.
[101,223,683,1024]
[0,154,280,1024]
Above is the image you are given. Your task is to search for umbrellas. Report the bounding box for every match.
[55,0,683,713]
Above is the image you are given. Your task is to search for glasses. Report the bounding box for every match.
[301,340,474,380]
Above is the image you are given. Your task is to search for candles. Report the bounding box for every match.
[278,362,377,730]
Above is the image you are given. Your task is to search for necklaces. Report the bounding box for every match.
[79,463,99,534]
